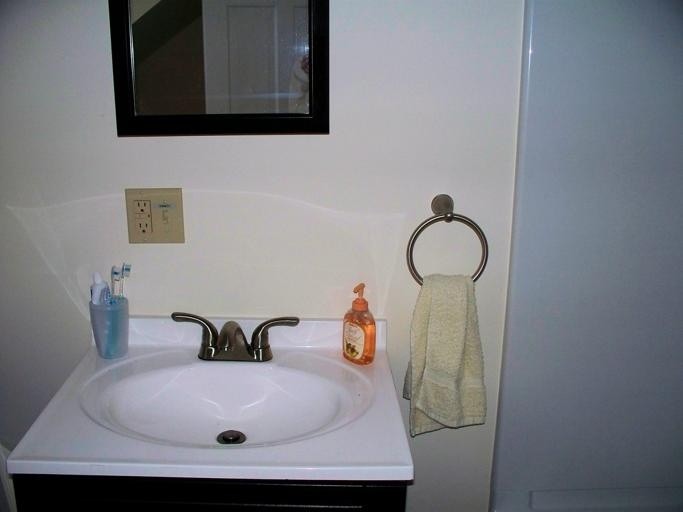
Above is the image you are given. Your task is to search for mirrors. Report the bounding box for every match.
[107,0,331,137]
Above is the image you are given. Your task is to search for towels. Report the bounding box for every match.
[402,282,486,436]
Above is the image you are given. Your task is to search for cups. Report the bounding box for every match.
[89,296,129,359]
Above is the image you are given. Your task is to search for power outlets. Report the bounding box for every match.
[124,187,184,246]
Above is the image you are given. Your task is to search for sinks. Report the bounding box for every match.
[5,314,416,483]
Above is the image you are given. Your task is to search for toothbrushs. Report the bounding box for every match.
[104,265,120,357]
[112,261,132,357]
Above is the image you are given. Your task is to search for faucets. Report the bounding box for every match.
[171,311,300,364]
